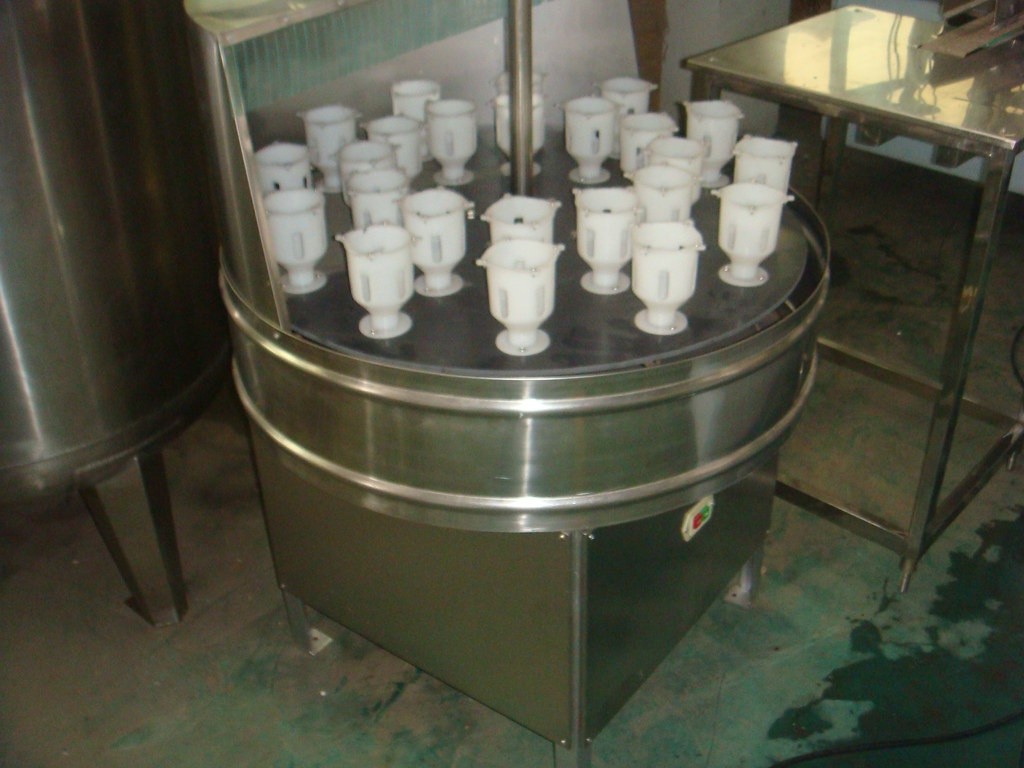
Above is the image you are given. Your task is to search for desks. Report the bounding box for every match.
[677,5,1024,591]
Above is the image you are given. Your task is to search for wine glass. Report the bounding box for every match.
[253,72,797,359]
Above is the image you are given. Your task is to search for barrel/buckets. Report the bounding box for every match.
[0,2,235,513]
